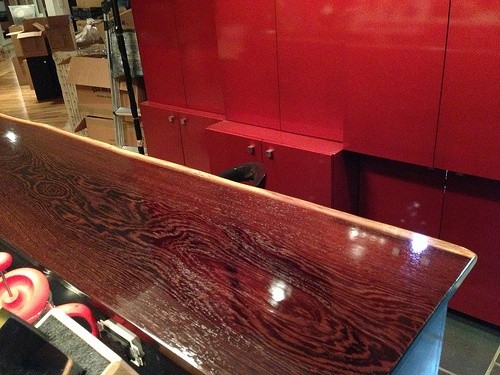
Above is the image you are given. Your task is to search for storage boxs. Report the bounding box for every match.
[7,0,145,147]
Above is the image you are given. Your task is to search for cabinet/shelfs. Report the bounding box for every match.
[131,1,500,330]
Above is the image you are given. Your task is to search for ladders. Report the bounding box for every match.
[101,0,148,154]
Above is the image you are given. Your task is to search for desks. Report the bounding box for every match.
[1,114,479,375]
